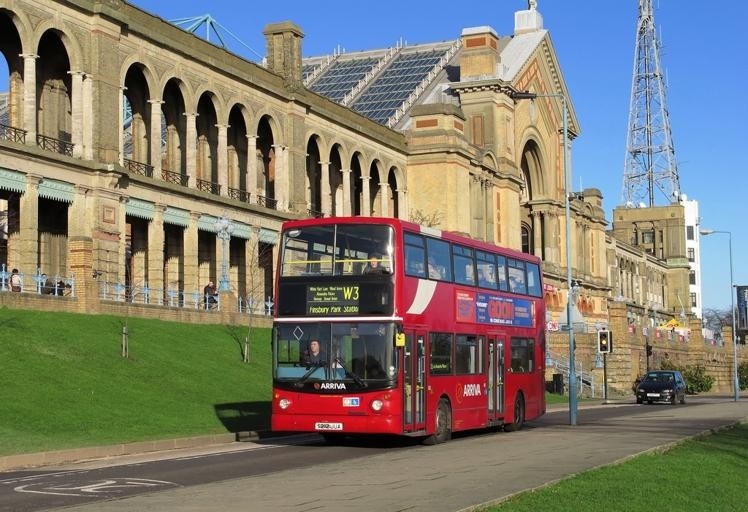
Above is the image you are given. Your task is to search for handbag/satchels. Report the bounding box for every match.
[12,286,21,292]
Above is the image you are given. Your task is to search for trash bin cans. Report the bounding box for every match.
[553,374,563,395]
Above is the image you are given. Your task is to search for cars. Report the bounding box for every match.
[635,369,688,406]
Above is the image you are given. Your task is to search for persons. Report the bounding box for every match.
[39,273,54,296]
[202,281,218,311]
[414,257,522,295]
[52,274,64,297]
[360,256,386,277]
[300,337,331,368]
[10,268,24,293]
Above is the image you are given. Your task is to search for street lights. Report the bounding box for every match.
[699,229,740,401]
[508,90,578,427]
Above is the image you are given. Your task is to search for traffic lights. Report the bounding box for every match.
[597,330,610,353]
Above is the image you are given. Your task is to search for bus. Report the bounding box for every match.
[269,216,549,447]
